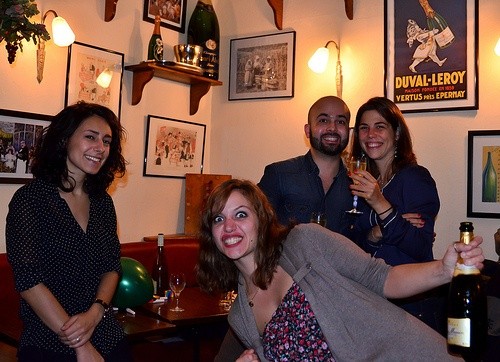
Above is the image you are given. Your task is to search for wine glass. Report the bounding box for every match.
[345,153,367,214]
[169,272,186,312]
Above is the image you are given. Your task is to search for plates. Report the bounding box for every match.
[165,60,203,74]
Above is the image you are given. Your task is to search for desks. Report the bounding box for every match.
[140,286,239,362]
[0,308,176,362]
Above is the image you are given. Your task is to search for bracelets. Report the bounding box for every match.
[378,206,392,216]
[94,298,110,313]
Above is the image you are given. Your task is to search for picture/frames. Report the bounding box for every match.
[143,115,206,179]
[384,0,479,114]
[228,30,296,101]
[65,41,124,125]
[467,130,500,219]
[143,0,187,34]
[0,109,55,184]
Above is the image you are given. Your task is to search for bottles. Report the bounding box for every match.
[187,0,220,81]
[152,233,169,299]
[447,222,487,357]
[148,15,163,62]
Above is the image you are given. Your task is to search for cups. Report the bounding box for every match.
[174,44,203,67]
[310,211,326,228]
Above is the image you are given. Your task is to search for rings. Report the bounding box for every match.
[76,337,80,342]
[453,242,458,252]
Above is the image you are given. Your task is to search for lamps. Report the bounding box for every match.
[36,9,76,84]
[308,41,343,101]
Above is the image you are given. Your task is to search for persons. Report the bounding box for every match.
[347,97,440,314]
[193,178,484,362]
[0,139,35,174]
[5,100,126,362]
[256,96,359,242]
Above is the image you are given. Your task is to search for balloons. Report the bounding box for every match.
[111,256,154,310]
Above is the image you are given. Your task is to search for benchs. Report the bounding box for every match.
[0,238,231,362]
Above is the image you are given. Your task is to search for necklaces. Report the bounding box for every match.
[249,288,259,306]
